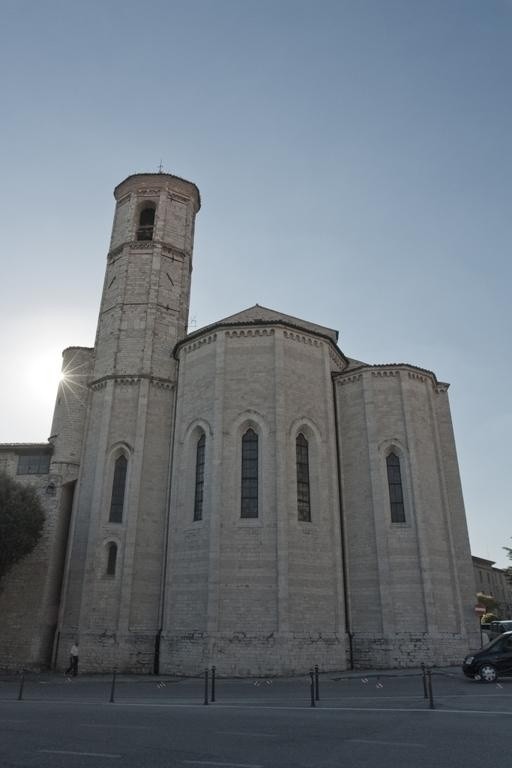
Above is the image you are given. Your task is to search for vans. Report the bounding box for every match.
[462,630,512,682]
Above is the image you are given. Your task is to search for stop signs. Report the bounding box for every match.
[473,603,486,616]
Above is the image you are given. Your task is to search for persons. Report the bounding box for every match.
[63,642,80,678]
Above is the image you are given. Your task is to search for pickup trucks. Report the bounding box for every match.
[480,620,512,640]
